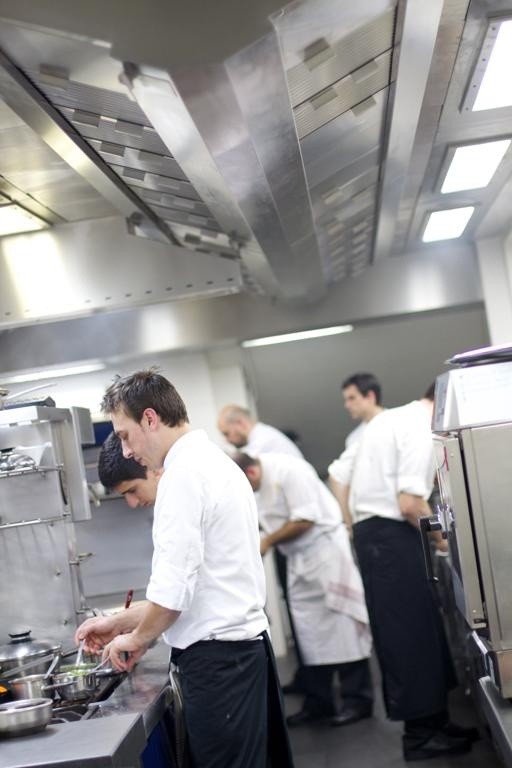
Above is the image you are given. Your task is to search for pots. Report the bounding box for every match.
[0,624,116,704]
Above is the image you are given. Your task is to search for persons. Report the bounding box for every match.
[319,377,484,762]
[216,448,380,730]
[216,403,309,700]
[72,363,304,768]
[97,429,163,511]
[339,370,394,449]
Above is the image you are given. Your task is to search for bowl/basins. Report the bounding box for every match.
[0,698,52,737]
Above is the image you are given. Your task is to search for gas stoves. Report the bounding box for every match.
[0,660,139,723]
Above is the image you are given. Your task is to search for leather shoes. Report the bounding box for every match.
[286,710,326,729]
[406,736,474,761]
[332,707,371,727]
[282,683,307,695]
[441,718,487,741]
